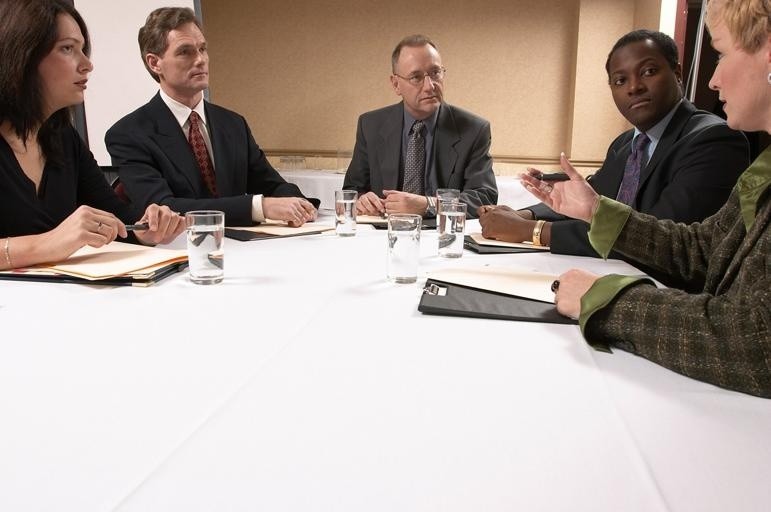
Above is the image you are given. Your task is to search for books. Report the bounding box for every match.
[0,240,189,287]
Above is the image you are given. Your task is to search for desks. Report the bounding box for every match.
[2,208,769,511]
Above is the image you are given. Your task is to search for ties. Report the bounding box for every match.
[402,123,426,196]
[616,136,649,208]
[187,112,216,199]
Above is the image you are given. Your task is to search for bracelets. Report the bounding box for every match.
[533,220,546,246]
[5,238,13,268]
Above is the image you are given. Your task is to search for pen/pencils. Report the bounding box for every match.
[536,173,569,180]
[125,222,149,230]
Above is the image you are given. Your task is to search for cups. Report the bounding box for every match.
[438,201,467,258]
[334,190,358,237]
[184,210,225,285]
[387,213,422,284]
[436,188,461,233]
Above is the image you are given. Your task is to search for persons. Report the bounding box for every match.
[477,29,752,259]
[0,0,185,272]
[104,7,322,227]
[342,36,498,220]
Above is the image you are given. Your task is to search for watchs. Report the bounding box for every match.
[424,195,437,220]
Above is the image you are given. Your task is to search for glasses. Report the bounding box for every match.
[397,68,446,84]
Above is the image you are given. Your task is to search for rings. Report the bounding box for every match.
[97,221,102,234]
[552,279,559,294]
[519,0,771,400]
[543,185,553,194]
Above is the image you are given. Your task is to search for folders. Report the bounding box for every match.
[222,229,323,242]
[372,216,438,231]
[417,278,580,326]
[462,235,550,254]
[0,255,190,288]
